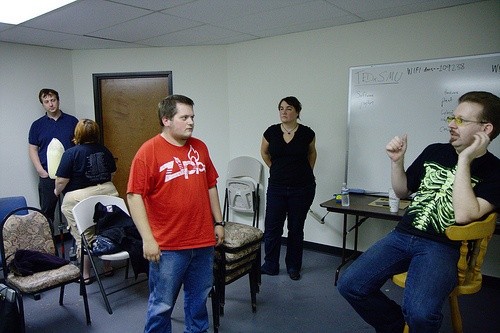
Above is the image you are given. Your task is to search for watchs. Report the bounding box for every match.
[215,222,225,227]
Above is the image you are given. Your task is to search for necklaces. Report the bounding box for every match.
[282,124,298,135]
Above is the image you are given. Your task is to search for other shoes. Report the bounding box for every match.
[260,264,268,274]
[287,270,300,280]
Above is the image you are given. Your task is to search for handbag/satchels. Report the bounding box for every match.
[3,249,70,277]
[0,284,19,333]
[93,235,122,254]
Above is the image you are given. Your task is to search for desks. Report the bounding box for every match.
[320,192,412,286]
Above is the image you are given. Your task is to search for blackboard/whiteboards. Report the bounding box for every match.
[345,52,500,202]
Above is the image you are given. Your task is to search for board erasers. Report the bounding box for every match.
[349,188,365,193]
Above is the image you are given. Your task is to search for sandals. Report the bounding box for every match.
[96,268,114,277]
[75,278,92,285]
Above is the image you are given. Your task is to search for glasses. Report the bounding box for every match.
[446,116,489,125]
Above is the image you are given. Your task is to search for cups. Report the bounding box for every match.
[389,188,400,212]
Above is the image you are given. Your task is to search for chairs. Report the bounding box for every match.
[222,155,263,230]
[207,221,264,332]
[393,213,496,333]
[0,195,130,326]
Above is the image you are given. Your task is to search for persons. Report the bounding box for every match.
[54,119,117,285]
[126,94,225,333]
[28,89,80,258]
[336,91,500,333]
[261,97,317,280]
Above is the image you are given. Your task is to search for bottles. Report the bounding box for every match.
[341,183,350,207]
